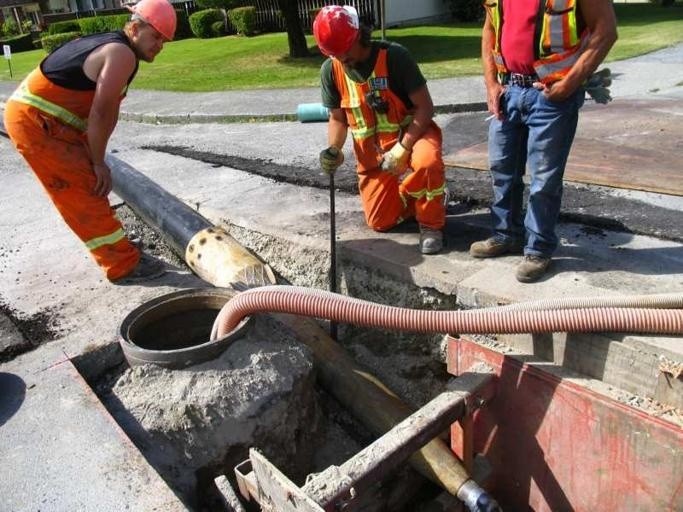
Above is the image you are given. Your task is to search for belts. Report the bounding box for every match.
[505,69,544,89]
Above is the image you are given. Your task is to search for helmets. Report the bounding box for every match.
[123,0,177,42]
[311,4,360,58]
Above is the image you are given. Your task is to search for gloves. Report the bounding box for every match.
[318,146,344,173]
[380,141,414,177]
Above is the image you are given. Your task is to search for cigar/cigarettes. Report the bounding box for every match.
[484,110,504,122]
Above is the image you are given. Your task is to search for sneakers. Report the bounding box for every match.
[417,228,443,254]
[111,257,163,283]
[469,233,512,259]
[516,254,551,283]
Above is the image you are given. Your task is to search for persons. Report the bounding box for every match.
[3,0,179,285]
[312,3,449,254]
[470,1,617,283]
